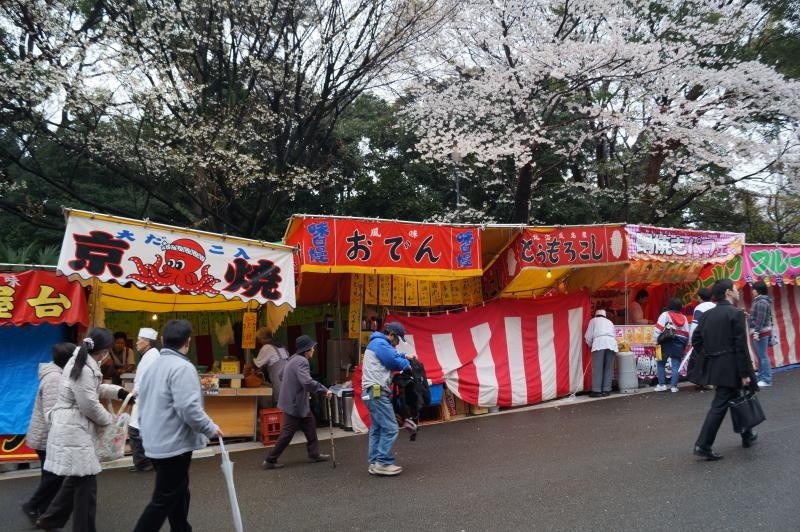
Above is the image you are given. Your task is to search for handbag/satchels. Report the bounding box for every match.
[657,327,673,343]
[93,413,129,462]
[728,385,766,433]
[767,331,778,346]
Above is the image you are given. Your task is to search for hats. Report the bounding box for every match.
[138,327,158,341]
[595,310,607,316]
[52,343,76,364]
[295,335,318,354]
[385,322,406,343]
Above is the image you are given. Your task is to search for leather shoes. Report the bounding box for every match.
[742,432,758,447]
[694,445,723,459]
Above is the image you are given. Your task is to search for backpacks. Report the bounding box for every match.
[393,358,431,402]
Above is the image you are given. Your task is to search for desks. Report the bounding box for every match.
[99,387,273,442]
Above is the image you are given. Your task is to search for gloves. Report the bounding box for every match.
[753,332,760,341]
[118,389,136,406]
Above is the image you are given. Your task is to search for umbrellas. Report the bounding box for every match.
[219,435,243,532]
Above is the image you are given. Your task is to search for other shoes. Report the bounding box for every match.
[589,391,603,397]
[697,384,714,390]
[368,462,403,476]
[262,460,284,468]
[129,463,153,472]
[602,391,609,396]
[311,454,329,462]
[758,380,773,387]
[671,387,679,393]
[654,385,667,391]
[22,502,39,523]
[36,516,63,532]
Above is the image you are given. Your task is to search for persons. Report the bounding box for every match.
[35,326,137,532]
[134,318,224,532]
[362,322,413,475]
[128,327,160,471]
[690,288,717,390]
[253,332,289,397]
[263,335,332,467]
[101,332,135,385]
[745,282,773,386]
[630,289,654,324]
[22,343,76,523]
[585,309,619,397]
[691,279,759,460]
[653,298,688,392]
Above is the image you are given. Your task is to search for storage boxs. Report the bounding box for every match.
[258,408,284,446]
